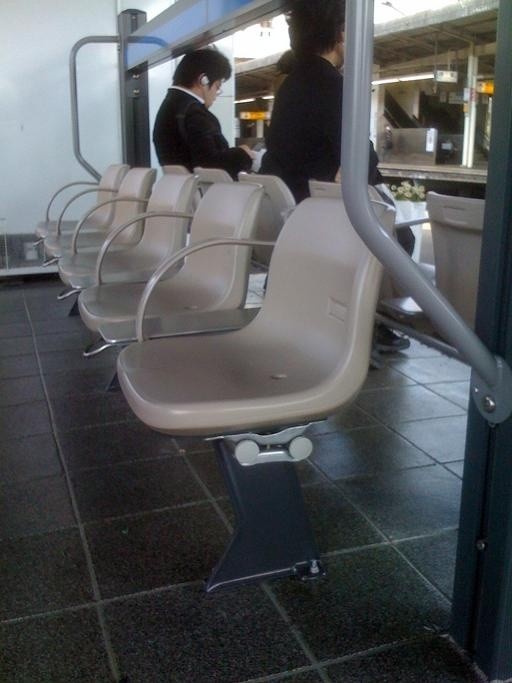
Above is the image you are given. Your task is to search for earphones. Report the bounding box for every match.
[200,75,210,87]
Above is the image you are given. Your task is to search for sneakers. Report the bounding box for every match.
[373,322,410,351]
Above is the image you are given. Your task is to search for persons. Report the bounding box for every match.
[260,1,415,349]
[152,49,256,181]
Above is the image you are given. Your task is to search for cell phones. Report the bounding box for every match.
[250,141,263,153]
